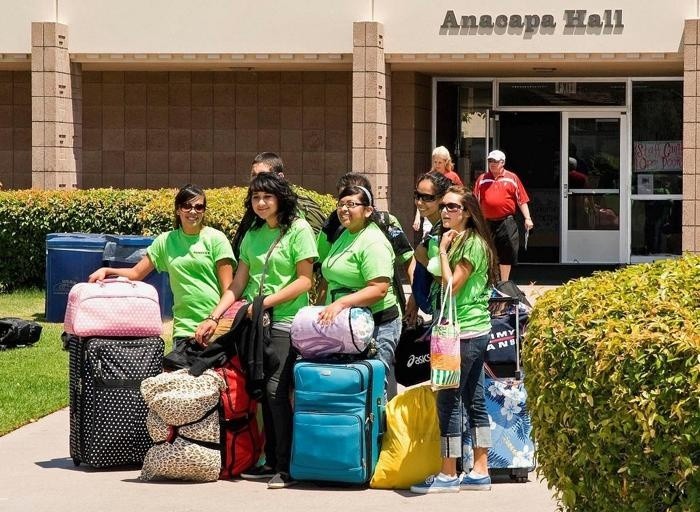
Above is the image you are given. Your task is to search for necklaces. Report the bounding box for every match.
[440,231,467,306]
[326,228,366,269]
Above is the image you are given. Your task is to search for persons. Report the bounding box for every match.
[473,150,534,282]
[88,184,238,349]
[317,173,417,401]
[195,171,321,488]
[404,170,454,325]
[317,185,402,401]
[410,185,501,492]
[231,152,328,260]
[568,143,682,252]
[412,146,463,238]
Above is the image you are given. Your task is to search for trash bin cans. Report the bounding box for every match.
[43,233,174,323]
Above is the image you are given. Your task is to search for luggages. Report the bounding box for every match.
[61,331,165,470]
[289,356,387,486]
[460,297,536,484]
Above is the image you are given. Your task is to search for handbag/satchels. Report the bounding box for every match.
[393,316,518,388]
[427,322,461,394]
[1,317,42,346]
[139,350,266,483]
[63,276,164,338]
[209,300,251,346]
[288,306,376,361]
[369,379,442,490]
[412,235,443,316]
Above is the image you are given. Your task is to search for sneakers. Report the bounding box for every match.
[458,474,492,492]
[410,473,461,494]
[241,463,275,479]
[268,471,297,489]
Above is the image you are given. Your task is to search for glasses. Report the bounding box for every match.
[438,202,465,213]
[335,202,368,209]
[413,190,440,202]
[178,202,206,214]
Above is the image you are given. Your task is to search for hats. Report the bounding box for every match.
[487,150,507,162]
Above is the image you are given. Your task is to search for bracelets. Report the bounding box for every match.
[525,218,531,220]
[439,252,446,255]
[206,316,219,323]
